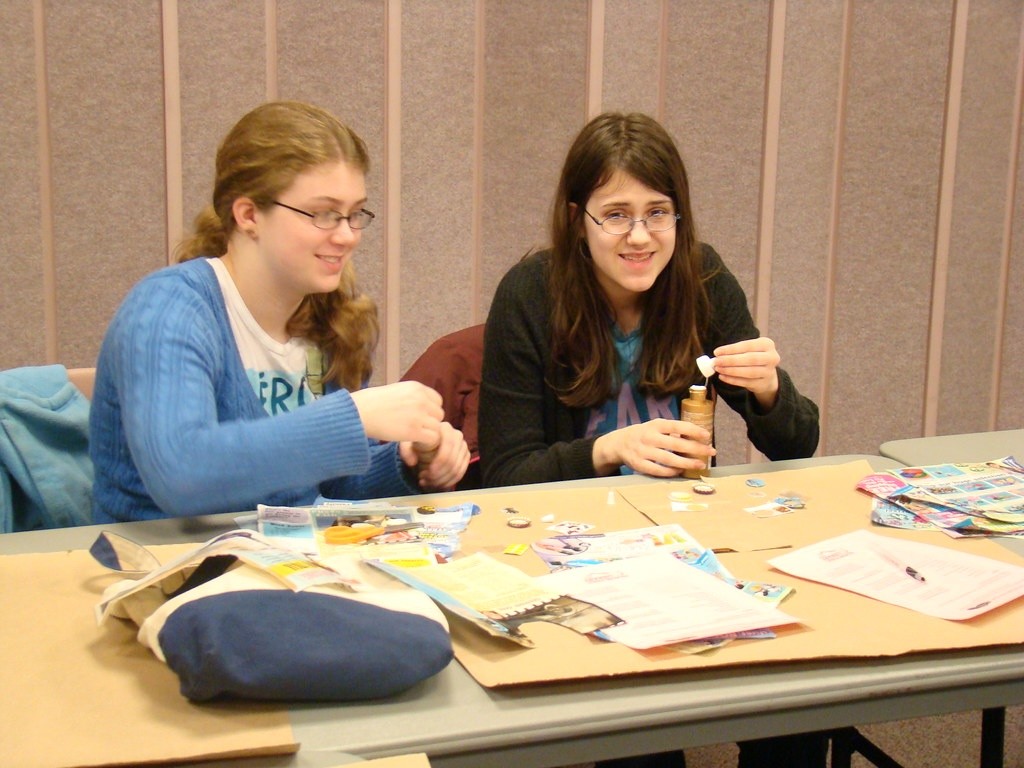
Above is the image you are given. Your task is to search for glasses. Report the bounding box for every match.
[582,206,680,235]
[270,200,376,230]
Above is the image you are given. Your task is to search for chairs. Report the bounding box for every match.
[0,363,97,534]
[397,323,485,465]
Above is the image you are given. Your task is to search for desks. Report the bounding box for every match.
[0,427,1024,768]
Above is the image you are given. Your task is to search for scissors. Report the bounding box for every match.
[324,522,424,545]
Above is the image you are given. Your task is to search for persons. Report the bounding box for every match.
[87,101,471,526]
[477,111,831,768]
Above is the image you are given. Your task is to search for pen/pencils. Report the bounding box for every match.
[882,551,927,582]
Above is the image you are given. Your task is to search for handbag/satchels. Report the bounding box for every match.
[91,528,453,703]
